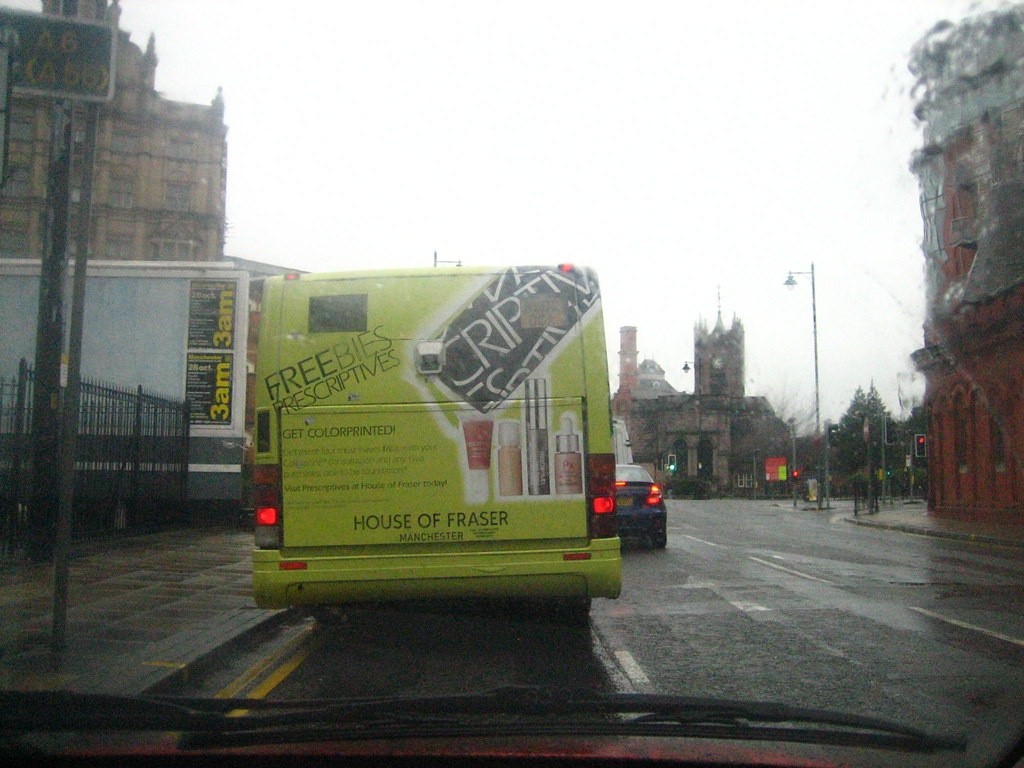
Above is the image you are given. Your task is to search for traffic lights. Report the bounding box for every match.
[914,433,926,457]
[885,416,896,445]
[791,469,799,478]
[823,418,839,449]
[662,454,677,471]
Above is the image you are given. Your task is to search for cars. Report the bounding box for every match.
[615,462,667,547]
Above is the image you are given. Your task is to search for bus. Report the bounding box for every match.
[612,417,635,465]
[244,260,624,624]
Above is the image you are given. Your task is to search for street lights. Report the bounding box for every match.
[682,358,703,480]
[781,262,824,508]
